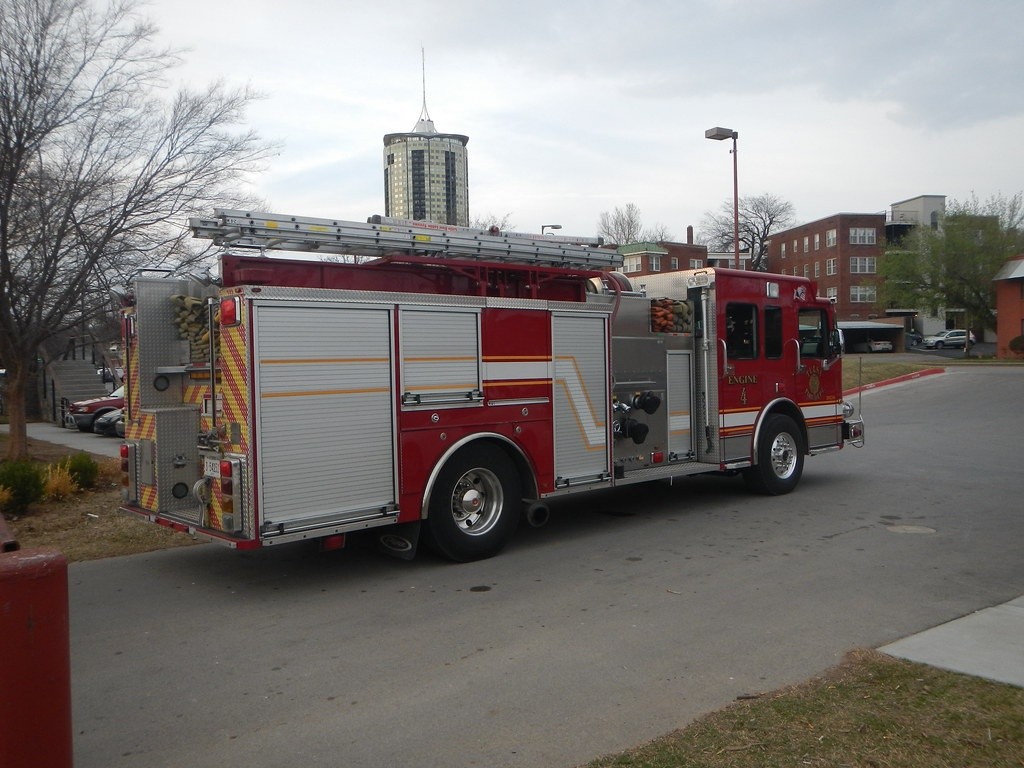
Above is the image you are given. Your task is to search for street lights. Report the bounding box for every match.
[705,127,739,270]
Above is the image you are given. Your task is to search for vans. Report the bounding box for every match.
[798,325,845,360]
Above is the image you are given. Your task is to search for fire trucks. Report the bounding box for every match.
[119,207,867,564]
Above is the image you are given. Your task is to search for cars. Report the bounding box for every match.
[904,332,922,346]
[93,409,121,437]
[852,336,892,353]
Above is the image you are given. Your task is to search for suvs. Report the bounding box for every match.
[922,329,976,350]
[65,385,124,433]
